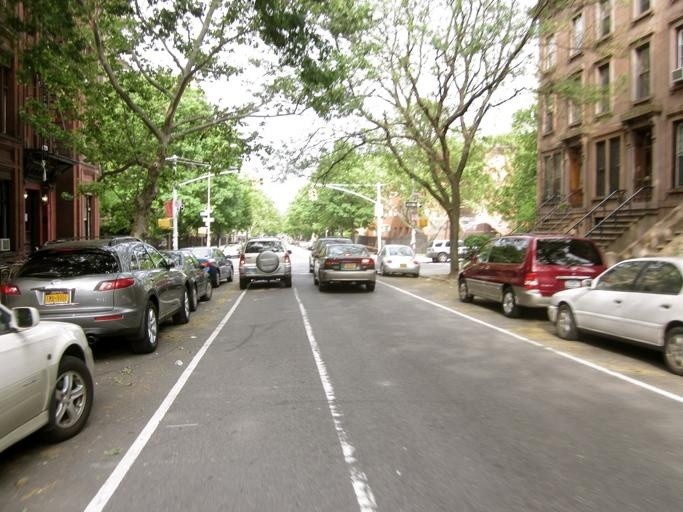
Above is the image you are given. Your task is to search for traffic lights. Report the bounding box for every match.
[308,189,318,203]
[159,217,174,232]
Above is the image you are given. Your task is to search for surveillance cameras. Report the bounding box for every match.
[179,203,184,209]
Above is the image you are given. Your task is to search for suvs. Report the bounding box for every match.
[238,237,293,289]
[0,237,194,357]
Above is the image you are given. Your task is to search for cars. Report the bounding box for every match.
[306,237,419,293]
[0,281,95,461]
[157,250,217,311]
[426,232,682,377]
[178,246,235,288]
